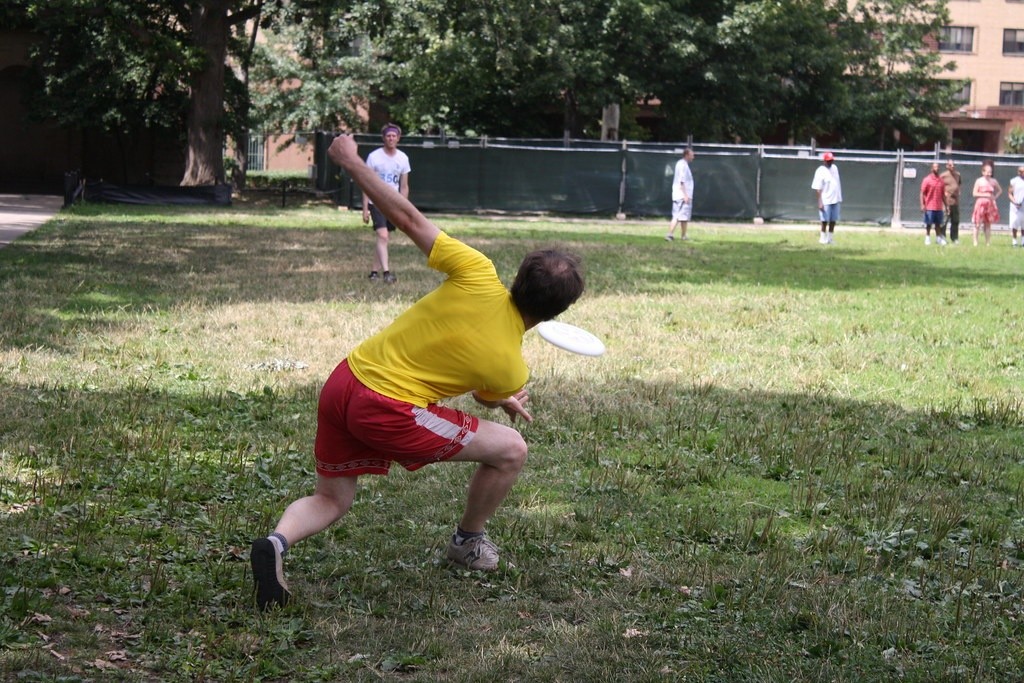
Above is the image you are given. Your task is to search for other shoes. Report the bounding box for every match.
[1012,239,1017,246]
[954,239,960,245]
[821,239,834,244]
[1021,237,1024,246]
[925,235,931,245]
[385,275,394,282]
[936,236,947,245]
[368,274,377,280]
[667,235,673,241]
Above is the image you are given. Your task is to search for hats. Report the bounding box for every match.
[823,153,833,160]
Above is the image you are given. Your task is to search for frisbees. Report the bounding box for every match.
[536,320,606,356]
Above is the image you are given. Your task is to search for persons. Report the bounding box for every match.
[970,160,1002,246]
[362,124,411,282]
[939,158,962,243]
[1007,166,1024,247]
[664,149,694,241]
[920,163,946,245]
[811,152,842,244]
[249,134,586,611]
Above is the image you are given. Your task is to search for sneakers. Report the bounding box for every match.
[447,533,514,575]
[250,538,291,610]
